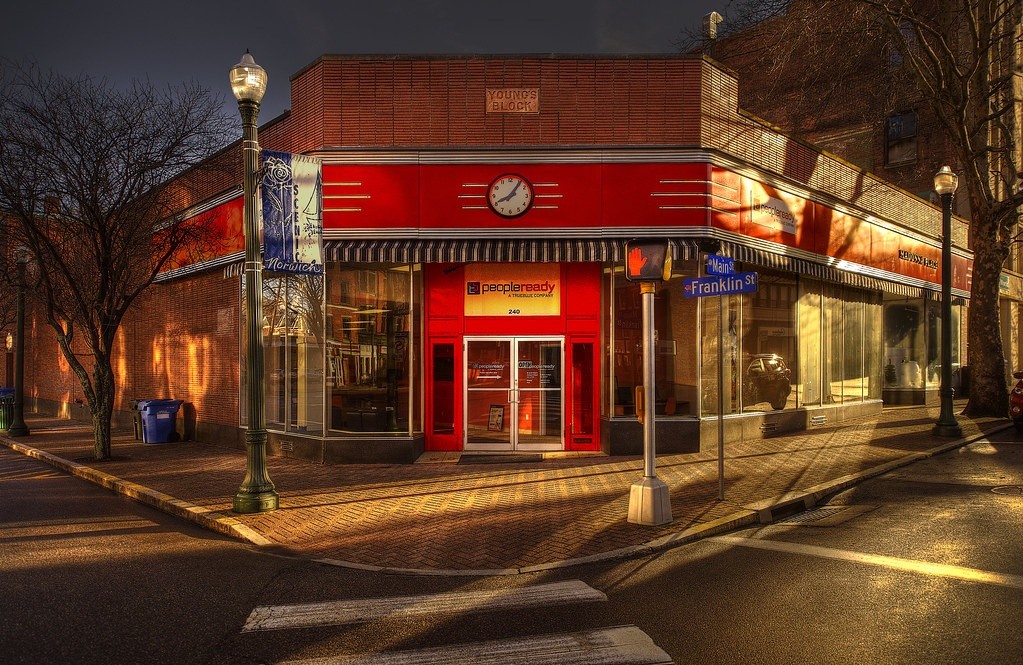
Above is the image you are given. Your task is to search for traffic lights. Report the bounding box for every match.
[625,238,673,281]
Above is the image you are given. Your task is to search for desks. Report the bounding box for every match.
[614,401,690,416]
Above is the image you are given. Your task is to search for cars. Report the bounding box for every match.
[265,367,308,384]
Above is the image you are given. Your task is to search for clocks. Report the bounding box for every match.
[487,173,534,218]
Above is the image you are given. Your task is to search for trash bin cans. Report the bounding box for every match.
[138,399,184,444]
[0,387,15,429]
[128,399,143,440]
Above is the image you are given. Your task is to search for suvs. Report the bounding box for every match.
[1010,371,1023,429]
[702,353,791,412]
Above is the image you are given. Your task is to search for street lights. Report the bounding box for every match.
[6,244,31,436]
[229,50,279,514]
[370,316,376,385]
[934,165,962,439]
